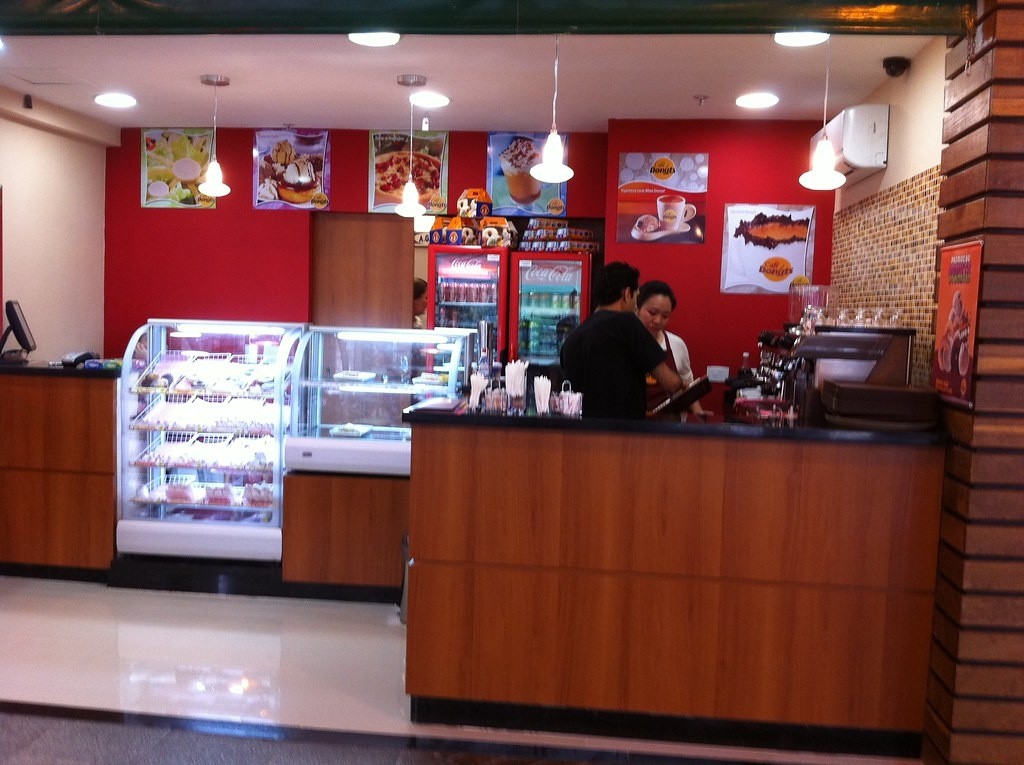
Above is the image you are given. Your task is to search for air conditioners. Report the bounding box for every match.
[810,102,891,191]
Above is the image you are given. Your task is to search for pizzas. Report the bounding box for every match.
[373,151,442,202]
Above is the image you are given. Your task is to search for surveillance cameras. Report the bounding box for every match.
[882,57,911,77]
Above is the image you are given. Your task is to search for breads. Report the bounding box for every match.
[636,215,659,232]
[142,370,279,507]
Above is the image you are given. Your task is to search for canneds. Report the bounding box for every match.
[437,281,498,328]
[518,290,580,354]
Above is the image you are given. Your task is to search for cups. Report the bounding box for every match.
[658,195,698,230]
[801,304,904,328]
[486,389,511,413]
[534,378,551,415]
[500,155,541,204]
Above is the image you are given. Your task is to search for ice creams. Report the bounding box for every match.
[266,136,323,205]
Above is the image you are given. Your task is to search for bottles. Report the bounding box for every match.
[740,352,752,379]
[400,356,413,383]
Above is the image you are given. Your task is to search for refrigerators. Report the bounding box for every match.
[426,244,508,373]
[509,252,591,366]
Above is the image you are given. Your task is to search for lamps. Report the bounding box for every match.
[337,385,423,394]
[348,32,401,47]
[395,75,427,220]
[174,323,285,336]
[197,74,232,198]
[772,29,831,47]
[529,34,575,184]
[332,331,454,345]
[799,36,847,190]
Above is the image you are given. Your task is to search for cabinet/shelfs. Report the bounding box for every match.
[114,319,473,604]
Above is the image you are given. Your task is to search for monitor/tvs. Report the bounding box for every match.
[0,301,36,355]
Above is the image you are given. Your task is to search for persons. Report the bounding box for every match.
[412,277,428,379]
[635,280,712,423]
[560,261,682,421]
